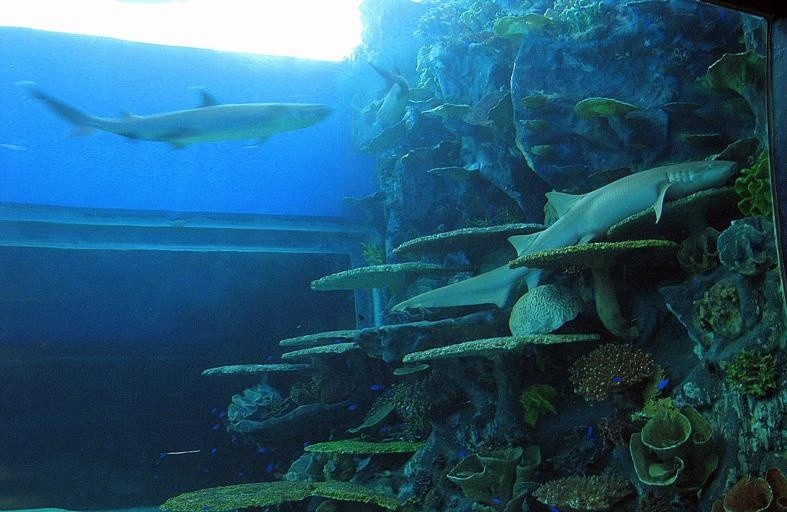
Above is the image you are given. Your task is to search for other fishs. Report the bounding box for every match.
[13,79,338,151]
